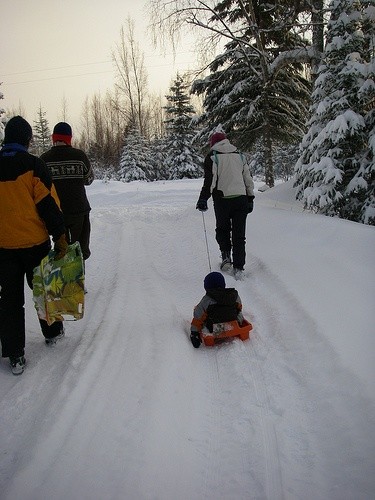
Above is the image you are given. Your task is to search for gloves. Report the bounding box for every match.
[196,198,207,211]
[52,234,68,260]
[247,197,253,213]
[190,331,202,349]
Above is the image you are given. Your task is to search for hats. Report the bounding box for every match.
[209,126,226,147]
[52,122,72,145]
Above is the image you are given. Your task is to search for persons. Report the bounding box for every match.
[196,132,255,279]
[0,116,68,376]
[40,122,95,260]
[190,272,243,348]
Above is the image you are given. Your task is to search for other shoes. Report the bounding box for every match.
[233,269,244,279]
[220,258,232,271]
[45,330,65,346]
[8,356,26,375]
